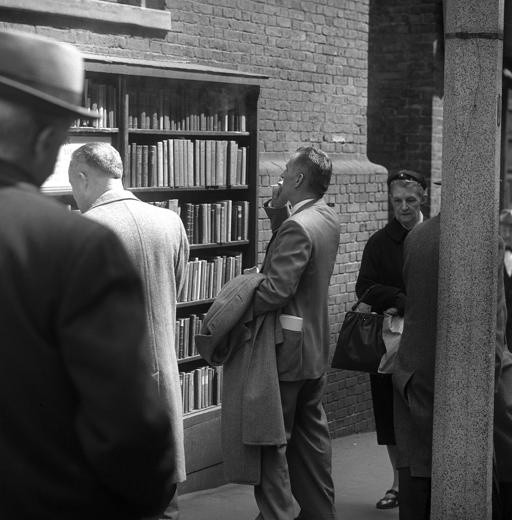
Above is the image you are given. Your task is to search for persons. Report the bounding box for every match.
[355,169,429,510]
[393,176,512,520]
[68,142,191,520]
[0,31,180,520]
[248,144,341,520]
[499,207,512,356]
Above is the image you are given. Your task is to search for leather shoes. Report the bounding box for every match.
[376,489,398,509]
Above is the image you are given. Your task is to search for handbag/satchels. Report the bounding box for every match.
[331,311,386,373]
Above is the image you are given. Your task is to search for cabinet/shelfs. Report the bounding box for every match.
[41,54,268,498]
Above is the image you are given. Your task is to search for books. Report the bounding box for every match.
[278,313,303,334]
[150,199,249,244]
[178,365,223,414]
[130,138,247,188]
[67,204,80,214]
[177,251,242,303]
[65,80,122,130]
[129,87,247,132]
[174,312,209,359]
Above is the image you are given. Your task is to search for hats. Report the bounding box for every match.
[387,170,427,190]
[0,29,100,120]
